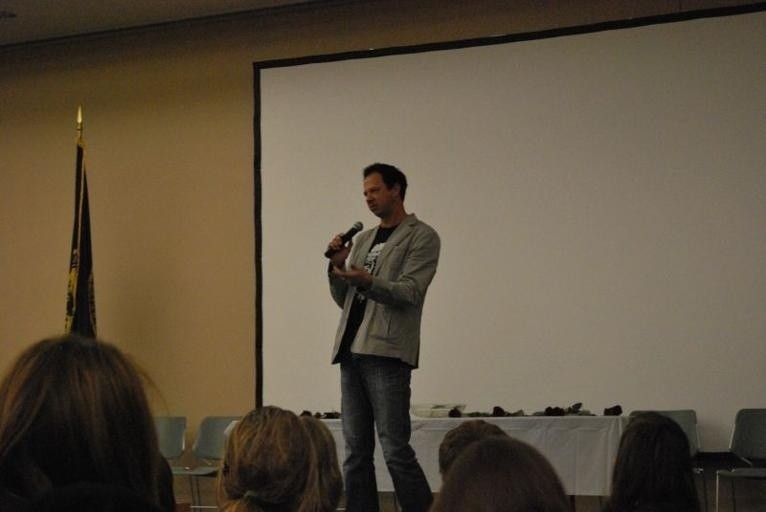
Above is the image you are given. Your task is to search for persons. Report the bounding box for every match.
[436,417,505,482]
[291,412,341,512]
[219,403,314,512]
[325,163,441,512]
[604,409,705,511]
[419,433,572,511]
[1,336,167,510]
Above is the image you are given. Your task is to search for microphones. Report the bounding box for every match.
[325,221,363,258]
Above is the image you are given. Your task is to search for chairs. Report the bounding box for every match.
[659,407,766,512]
[152,414,241,511]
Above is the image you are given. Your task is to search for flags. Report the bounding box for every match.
[54,137,98,344]
